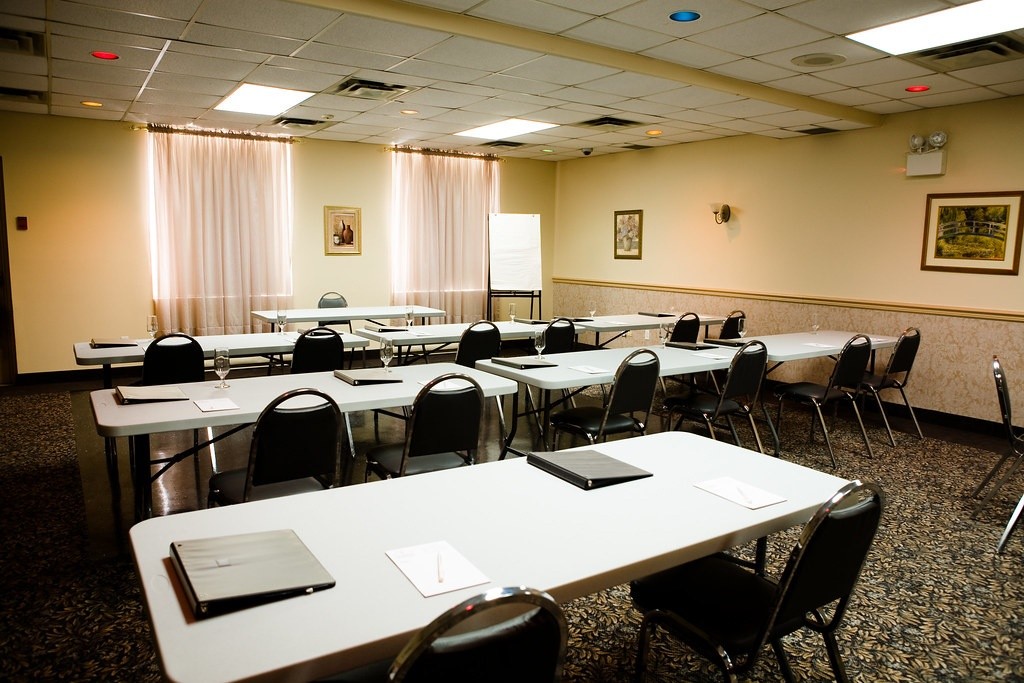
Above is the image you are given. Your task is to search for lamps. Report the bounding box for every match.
[708,200,730,224]
[905,128,950,176]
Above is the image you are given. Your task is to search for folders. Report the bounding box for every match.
[298,328,343,336]
[527,449,653,490]
[170,529,336,618]
[365,324,409,333]
[704,338,757,347]
[491,357,558,369]
[554,316,594,322]
[665,342,719,350]
[334,369,403,386]
[116,386,189,405]
[638,312,675,317]
[91,339,138,347]
[514,318,551,325]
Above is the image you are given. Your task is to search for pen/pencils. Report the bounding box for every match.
[437,553,444,583]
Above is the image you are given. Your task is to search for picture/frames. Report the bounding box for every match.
[920,191,1024,275]
[325,207,363,256]
[612,210,643,260]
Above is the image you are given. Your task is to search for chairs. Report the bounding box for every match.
[630,480,887,683]
[384,586,569,683]
[107,293,924,484]
[963,355,1024,518]
[207,389,356,509]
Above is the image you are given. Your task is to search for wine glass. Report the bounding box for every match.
[588,301,596,319]
[737,318,748,342]
[380,337,394,373]
[811,312,820,336]
[277,309,288,336]
[534,327,546,360]
[146,315,159,342]
[659,322,670,350]
[405,305,414,330]
[214,347,231,389]
[509,303,516,325]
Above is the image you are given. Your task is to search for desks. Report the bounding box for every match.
[474,341,769,460]
[730,329,899,457]
[250,304,445,324]
[90,362,522,521]
[355,319,585,367]
[128,424,857,683]
[74,325,370,389]
[557,311,729,351]
